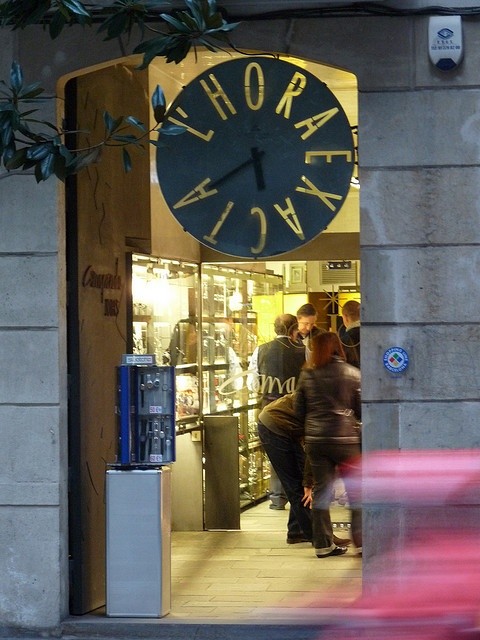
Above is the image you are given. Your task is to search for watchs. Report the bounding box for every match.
[153,372,160,391]
[165,416,171,460]
[140,372,145,408]
[162,371,168,409]
[140,419,147,461]
[160,416,166,455]
[146,374,154,392]
[148,417,153,455]
[153,417,159,454]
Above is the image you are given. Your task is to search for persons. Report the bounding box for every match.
[296,303,326,363]
[257,392,353,547]
[246,313,312,511]
[289,330,363,558]
[337,300,360,369]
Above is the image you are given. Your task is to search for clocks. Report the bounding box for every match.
[154,54,356,259]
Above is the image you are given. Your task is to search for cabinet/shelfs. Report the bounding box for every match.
[118,363,176,467]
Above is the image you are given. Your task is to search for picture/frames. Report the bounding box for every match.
[292,267,303,284]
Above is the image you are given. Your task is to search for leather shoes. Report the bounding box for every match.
[333,535,352,546]
[316,546,348,558]
